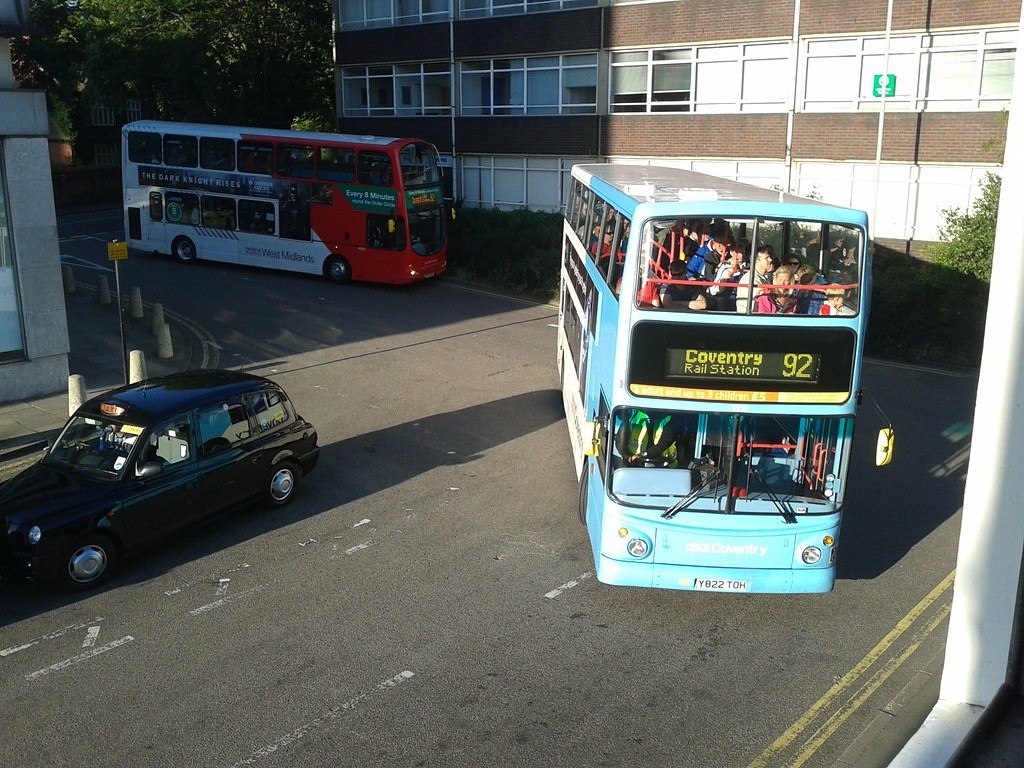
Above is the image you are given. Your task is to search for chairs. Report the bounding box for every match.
[156,433,191,464]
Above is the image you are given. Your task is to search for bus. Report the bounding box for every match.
[122,121,459,287]
[557,163,897,597]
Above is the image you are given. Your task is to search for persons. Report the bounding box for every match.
[98,422,138,457]
[573,193,854,318]
[200,142,427,186]
[610,407,792,468]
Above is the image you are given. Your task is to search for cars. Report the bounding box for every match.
[1,367,323,594]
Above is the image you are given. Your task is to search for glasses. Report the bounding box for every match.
[671,272,684,277]
[787,262,799,265]
[606,231,614,235]
